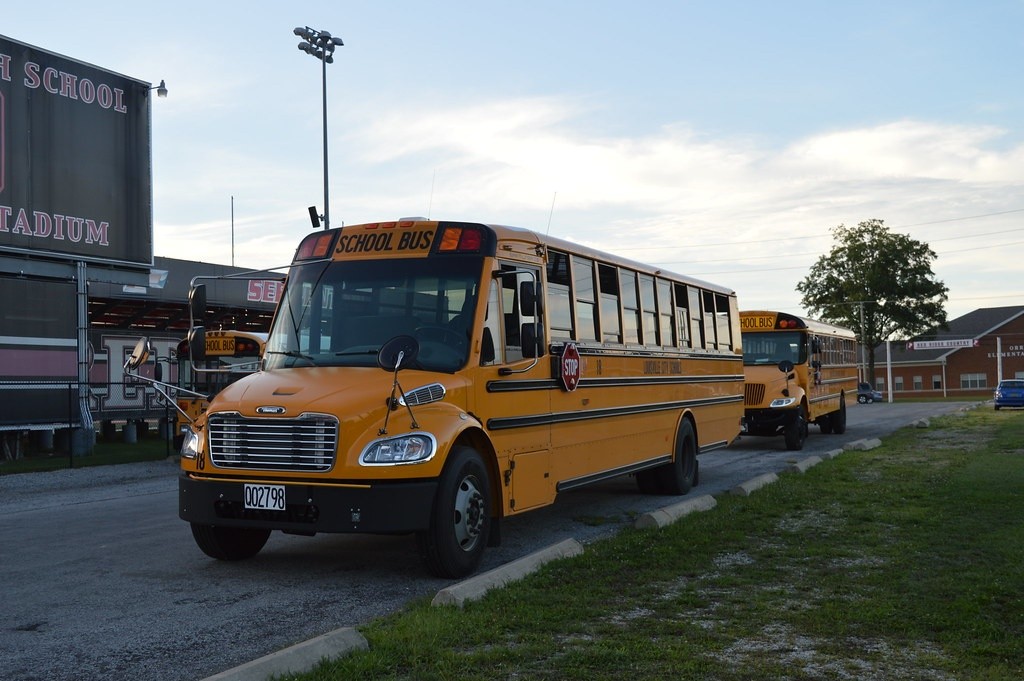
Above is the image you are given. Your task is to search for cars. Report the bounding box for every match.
[992,379,1024,411]
[867,390,883,403]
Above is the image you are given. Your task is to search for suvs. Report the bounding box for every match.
[858,381,874,404]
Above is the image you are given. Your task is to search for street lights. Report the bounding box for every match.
[290,25,346,230]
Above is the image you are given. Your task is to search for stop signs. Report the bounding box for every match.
[561,342,583,392]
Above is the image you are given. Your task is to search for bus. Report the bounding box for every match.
[123,216,748,582]
[719,310,860,450]
[153,328,331,449]
